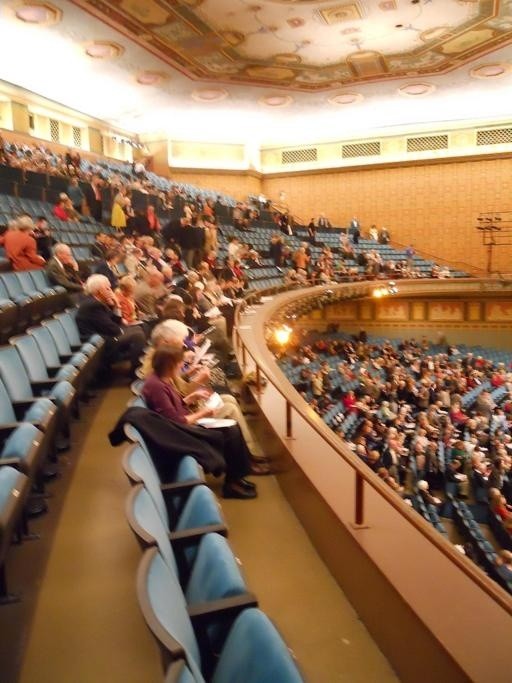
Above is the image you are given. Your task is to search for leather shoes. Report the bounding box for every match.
[222,482,257,499]
[246,463,273,476]
[232,477,256,490]
[249,453,271,464]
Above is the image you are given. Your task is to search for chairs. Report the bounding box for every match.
[126,479,231,591]
[121,338,303,452]
[1,383,48,518]
[123,442,214,532]
[1,464,38,604]
[212,226,472,293]
[276,332,511,470]
[2,139,238,207]
[408,471,510,589]
[1,195,110,459]
[136,526,258,657]
[163,607,304,682]
